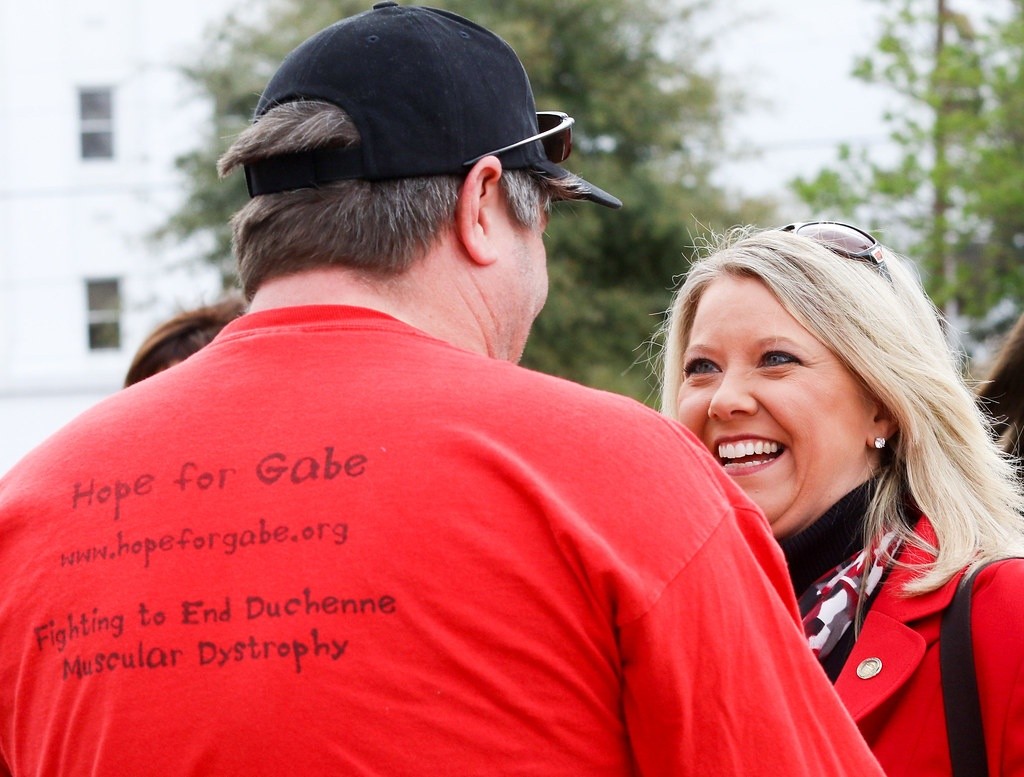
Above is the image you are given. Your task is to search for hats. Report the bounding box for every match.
[245,2,623,209]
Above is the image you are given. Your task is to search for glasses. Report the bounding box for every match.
[466,110,574,168]
[777,222,895,287]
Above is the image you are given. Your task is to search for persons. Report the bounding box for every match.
[652,223,1024,777]
[2,2,899,776]
